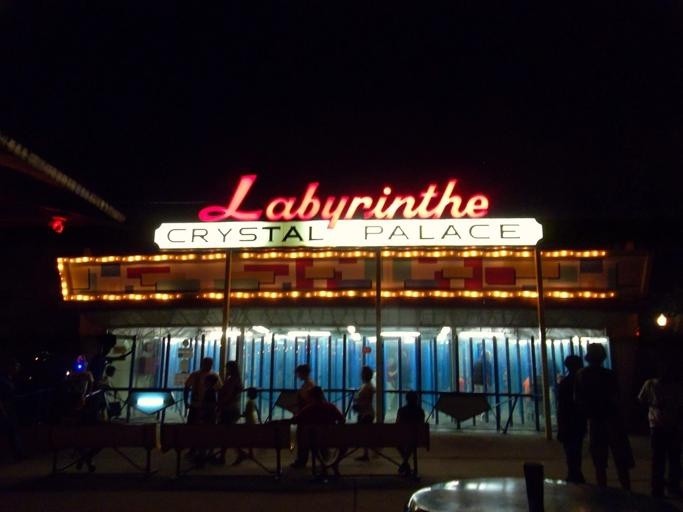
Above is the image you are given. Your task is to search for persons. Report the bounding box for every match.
[639,350,671,495]
[102,365,116,421]
[473,351,492,421]
[294,364,346,475]
[352,366,376,461]
[574,343,636,496]
[183,357,258,466]
[557,355,585,483]
[396,393,425,474]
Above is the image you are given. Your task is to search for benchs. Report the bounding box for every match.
[26,420,431,484]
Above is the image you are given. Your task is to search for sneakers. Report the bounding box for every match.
[233,451,248,466]
[398,462,410,473]
[288,462,305,468]
[76,461,84,469]
[210,457,226,465]
[355,455,368,460]
[88,464,96,472]
